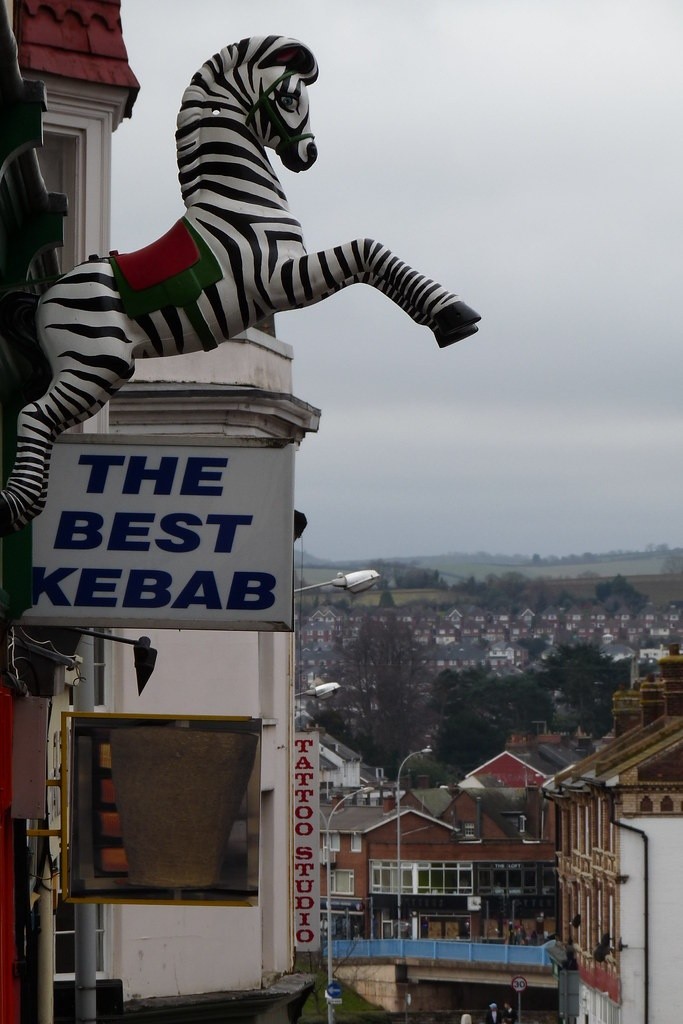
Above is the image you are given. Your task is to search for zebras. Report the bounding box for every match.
[0,33,482,532]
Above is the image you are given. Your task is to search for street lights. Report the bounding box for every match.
[320,786,375,986]
[397,746,433,940]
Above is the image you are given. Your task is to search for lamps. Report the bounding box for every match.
[47,624,158,696]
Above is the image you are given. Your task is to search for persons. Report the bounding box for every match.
[460,1003,516,1024]
[505,919,537,946]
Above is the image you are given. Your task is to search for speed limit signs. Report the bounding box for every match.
[512,976,528,991]
